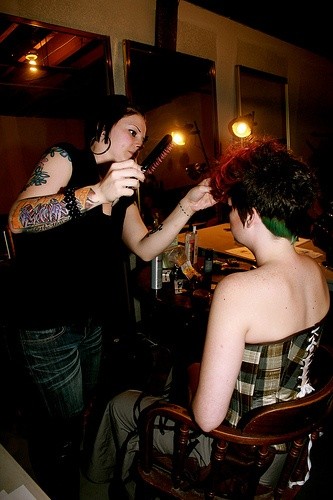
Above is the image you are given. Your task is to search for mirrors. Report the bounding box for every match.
[121,38,222,232]
[236,65,290,152]
[0,13,116,261]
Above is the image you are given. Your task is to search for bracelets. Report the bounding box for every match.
[64,186,82,219]
[179,203,192,217]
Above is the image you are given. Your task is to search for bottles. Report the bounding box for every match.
[151,253,163,293]
[169,267,184,290]
[204,248,213,275]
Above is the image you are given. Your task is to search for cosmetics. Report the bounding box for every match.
[205,249,212,287]
[185,226,198,264]
[151,254,162,290]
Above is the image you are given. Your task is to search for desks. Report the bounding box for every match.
[127,255,259,327]
[176,221,333,287]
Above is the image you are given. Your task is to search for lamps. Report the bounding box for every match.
[25,47,38,75]
[169,122,193,148]
[231,112,258,139]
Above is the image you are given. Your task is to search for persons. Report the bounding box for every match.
[8,94,219,427]
[85,137,330,486]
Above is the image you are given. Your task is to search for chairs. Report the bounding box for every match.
[135,385,333,500]
